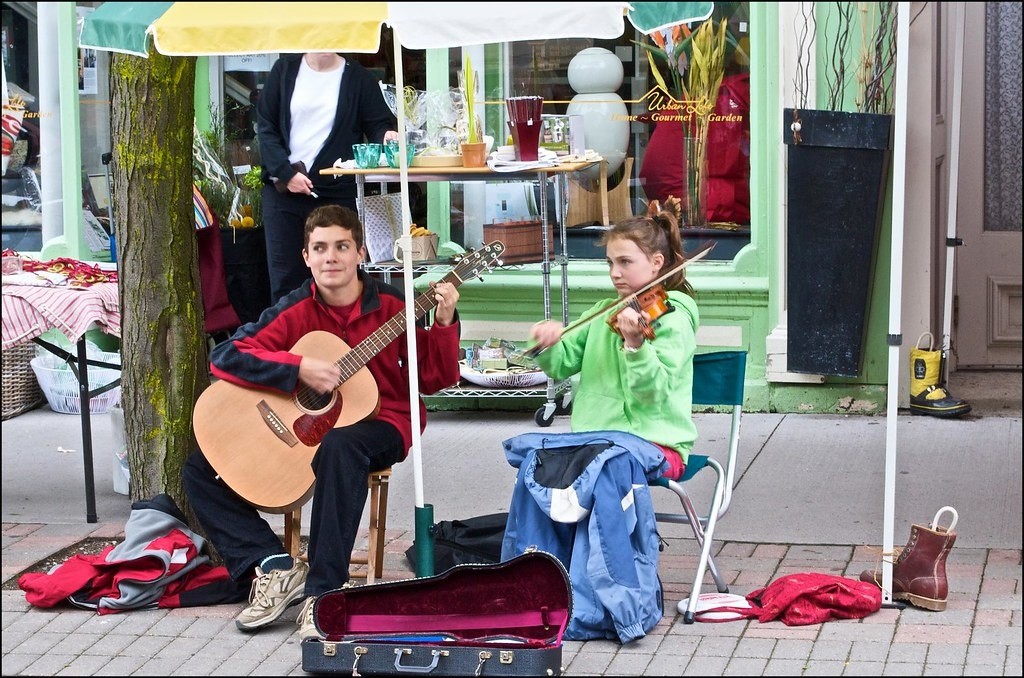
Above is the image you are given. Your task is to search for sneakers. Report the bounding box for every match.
[235,556,309,632]
[295,594,326,644]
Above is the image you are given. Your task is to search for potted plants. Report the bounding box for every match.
[459,58,486,168]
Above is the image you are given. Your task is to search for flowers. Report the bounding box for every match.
[631,20,729,227]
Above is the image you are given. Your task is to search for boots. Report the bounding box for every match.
[858,523,959,611]
[910,348,971,416]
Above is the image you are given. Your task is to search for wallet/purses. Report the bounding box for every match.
[274,160,306,193]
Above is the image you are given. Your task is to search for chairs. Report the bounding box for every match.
[650,351,749,624]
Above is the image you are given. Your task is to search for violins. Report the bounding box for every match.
[605,282,668,341]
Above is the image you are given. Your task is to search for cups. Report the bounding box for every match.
[383,145,415,169]
[351,143,382,169]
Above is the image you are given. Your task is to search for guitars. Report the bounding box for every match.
[192,239,505,515]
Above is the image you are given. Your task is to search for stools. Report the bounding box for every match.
[283,467,393,586]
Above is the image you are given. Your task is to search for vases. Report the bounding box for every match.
[682,136,706,227]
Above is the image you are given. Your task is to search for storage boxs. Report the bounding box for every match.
[413,234,438,262]
[484,220,556,267]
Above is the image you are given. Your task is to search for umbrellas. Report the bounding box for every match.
[76,2,714,508]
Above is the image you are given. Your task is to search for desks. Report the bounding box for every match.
[317,147,608,426]
[1,262,121,524]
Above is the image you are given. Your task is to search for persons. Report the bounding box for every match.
[256,53,407,308]
[179,205,462,641]
[522,213,700,481]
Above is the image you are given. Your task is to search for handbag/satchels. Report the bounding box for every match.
[355,192,413,264]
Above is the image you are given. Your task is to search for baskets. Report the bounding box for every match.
[1,341,47,421]
